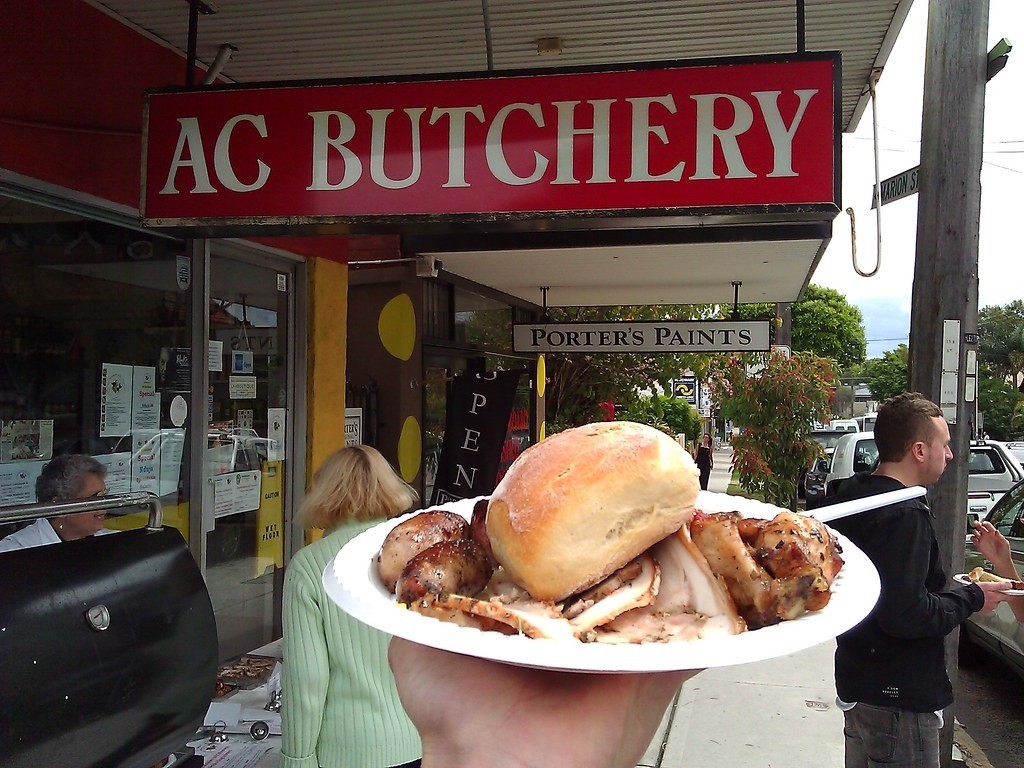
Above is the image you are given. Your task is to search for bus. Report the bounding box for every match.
[849,416,879,432]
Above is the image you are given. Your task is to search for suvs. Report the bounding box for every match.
[856,440,1020,543]
[799,429,856,496]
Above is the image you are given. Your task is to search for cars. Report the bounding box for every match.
[960,476,1024,679]
[805,448,836,509]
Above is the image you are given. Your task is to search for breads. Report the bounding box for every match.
[486,420,704,604]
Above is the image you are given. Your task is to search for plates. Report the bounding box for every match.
[323,487,882,676]
[953,572,1024,598]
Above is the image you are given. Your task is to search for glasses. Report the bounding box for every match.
[91,489,107,497]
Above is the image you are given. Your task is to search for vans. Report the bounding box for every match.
[830,419,860,434]
[822,432,881,497]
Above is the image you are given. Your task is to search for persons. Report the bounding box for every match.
[983,432,990,440]
[694,433,713,491]
[280,444,423,768]
[387,638,709,768]
[0,454,125,554]
[968,518,1024,626]
[818,391,1012,768]
